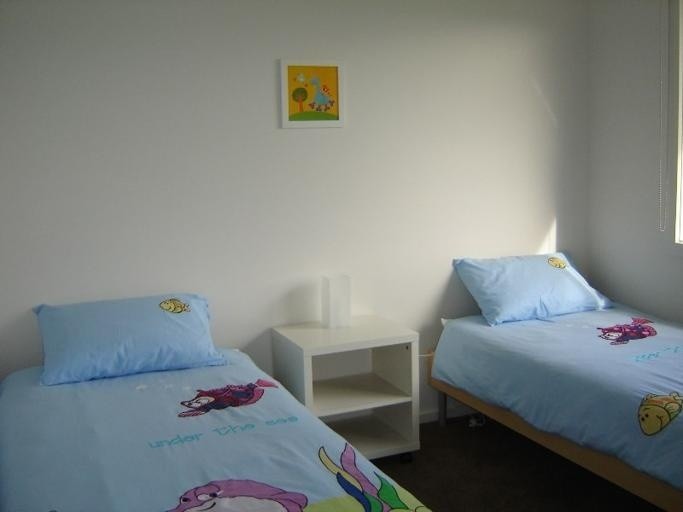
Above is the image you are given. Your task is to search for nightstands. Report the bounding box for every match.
[272,317,420,460]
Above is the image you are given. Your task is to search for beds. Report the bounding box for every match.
[427,303,683,512]
[0,350,431,512]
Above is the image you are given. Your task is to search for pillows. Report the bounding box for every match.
[453,252,617,326]
[32,292,230,386]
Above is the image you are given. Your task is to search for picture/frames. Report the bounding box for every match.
[280,58,345,128]
